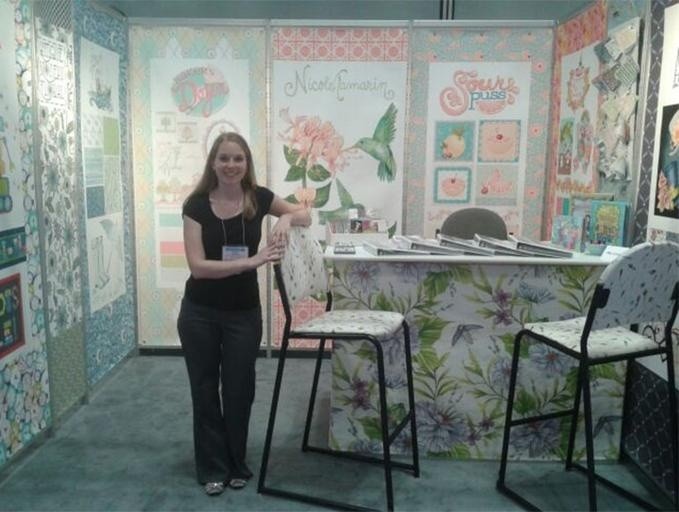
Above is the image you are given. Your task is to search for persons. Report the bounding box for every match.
[177,132,311,495]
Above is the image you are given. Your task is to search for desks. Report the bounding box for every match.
[314,234,627,462]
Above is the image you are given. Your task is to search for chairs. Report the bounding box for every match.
[495,238,679,512]
[256,223,422,512]
[442,208,507,244]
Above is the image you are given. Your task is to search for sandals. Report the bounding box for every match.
[229,477,247,488]
[204,480,226,495]
